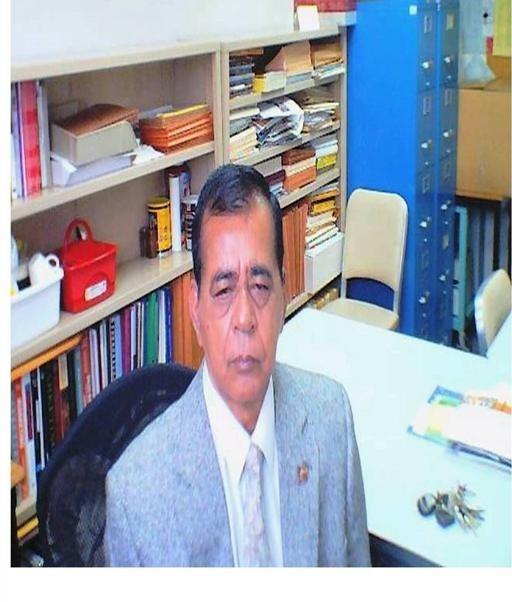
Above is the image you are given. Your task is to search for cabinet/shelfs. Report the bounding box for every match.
[347,0,460,346]
[221,25,348,319]
[11,42,223,523]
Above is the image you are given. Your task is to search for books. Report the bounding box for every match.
[229,40,344,161]
[11,285,172,534]
[254,146,340,304]
[408,385,512,462]
[11,78,214,201]
[305,286,339,310]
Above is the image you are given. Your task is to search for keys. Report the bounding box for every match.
[413,481,488,533]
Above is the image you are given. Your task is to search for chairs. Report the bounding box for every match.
[36,363,197,567]
[320,189,408,332]
[473,269,512,356]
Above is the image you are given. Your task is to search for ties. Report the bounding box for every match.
[237,442,281,568]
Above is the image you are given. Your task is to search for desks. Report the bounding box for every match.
[274,308,512,567]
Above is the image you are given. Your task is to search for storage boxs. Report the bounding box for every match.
[456,80,512,201]
[11,267,64,353]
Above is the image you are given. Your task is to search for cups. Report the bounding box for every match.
[28,252,60,286]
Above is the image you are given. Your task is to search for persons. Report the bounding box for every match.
[99,163,371,566]
